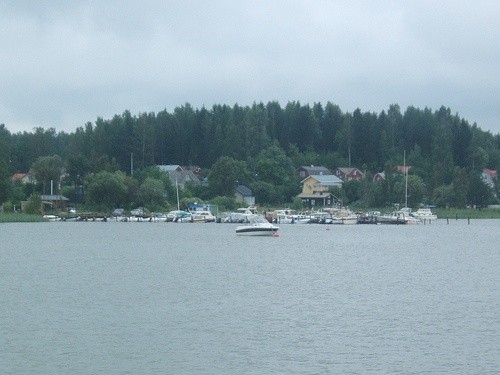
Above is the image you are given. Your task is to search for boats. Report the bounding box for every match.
[44,207,438,227]
[235,214,279,237]
